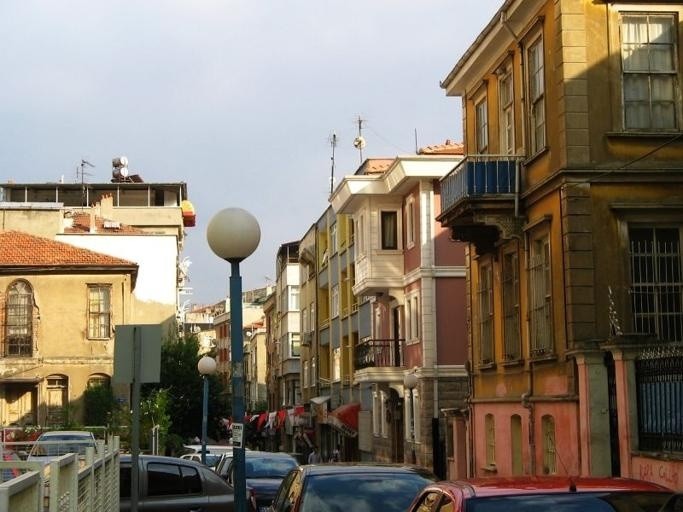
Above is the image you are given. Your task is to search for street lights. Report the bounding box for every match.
[206,206,261,512]
[195,354,218,465]
[403,375,419,464]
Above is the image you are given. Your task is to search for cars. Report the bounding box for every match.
[264,466,439,511]
[182,443,302,501]
[24,429,97,473]
[115,452,258,511]
[0,447,29,483]
[405,476,682,512]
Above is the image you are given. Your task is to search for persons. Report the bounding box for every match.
[307,448,321,465]
[300,442,309,465]
[327,445,340,464]
